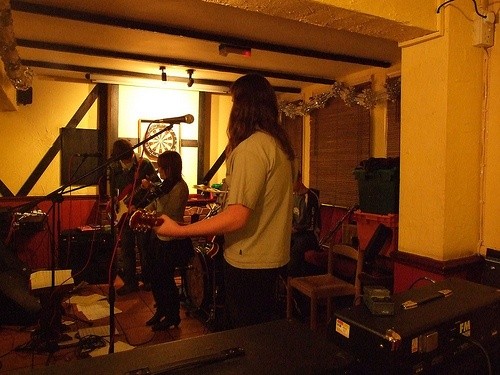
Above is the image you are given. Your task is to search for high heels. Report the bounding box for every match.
[146,311,164,326]
[153,312,181,331]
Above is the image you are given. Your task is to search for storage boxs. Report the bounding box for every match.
[352,168,401,258]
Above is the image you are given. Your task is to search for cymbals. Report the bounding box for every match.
[193,184,221,192]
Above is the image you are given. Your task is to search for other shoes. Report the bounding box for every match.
[143,281,151,290]
[116,282,139,294]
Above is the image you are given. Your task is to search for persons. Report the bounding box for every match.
[152,72,293,330]
[139,151,188,332]
[113,140,161,296]
[290,169,320,277]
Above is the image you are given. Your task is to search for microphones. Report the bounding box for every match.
[77,152,103,158]
[153,114,194,124]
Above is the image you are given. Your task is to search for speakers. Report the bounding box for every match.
[61,229,113,286]
[356,169,398,214]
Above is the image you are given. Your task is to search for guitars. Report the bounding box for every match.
[106,169,160,227]
[129,210,222,263]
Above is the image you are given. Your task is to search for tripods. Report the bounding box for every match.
[12,118,171,367]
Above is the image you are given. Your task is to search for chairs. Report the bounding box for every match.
[286,244,362,330]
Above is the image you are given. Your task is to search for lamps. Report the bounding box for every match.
[186,68,195,87]
[159,66,167,80]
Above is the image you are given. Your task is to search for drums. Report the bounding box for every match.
[181,247,223,314]
